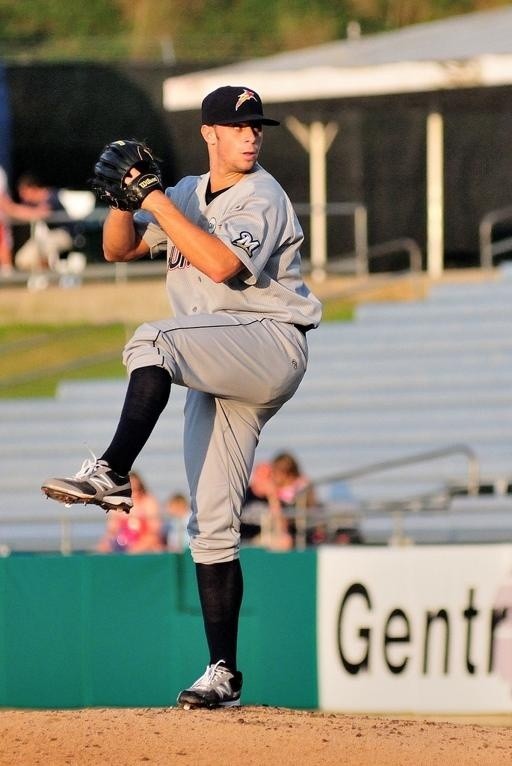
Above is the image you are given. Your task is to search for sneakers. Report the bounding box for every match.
[40,459,134,514]
[176,663,243,709]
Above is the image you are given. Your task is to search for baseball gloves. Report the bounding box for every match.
[92,139,164,209]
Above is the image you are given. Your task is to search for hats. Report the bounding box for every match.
[201,86,280,126]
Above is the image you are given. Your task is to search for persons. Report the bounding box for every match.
[0,165,88,289]
[92,453,364,555]
[41,84,324,712]
